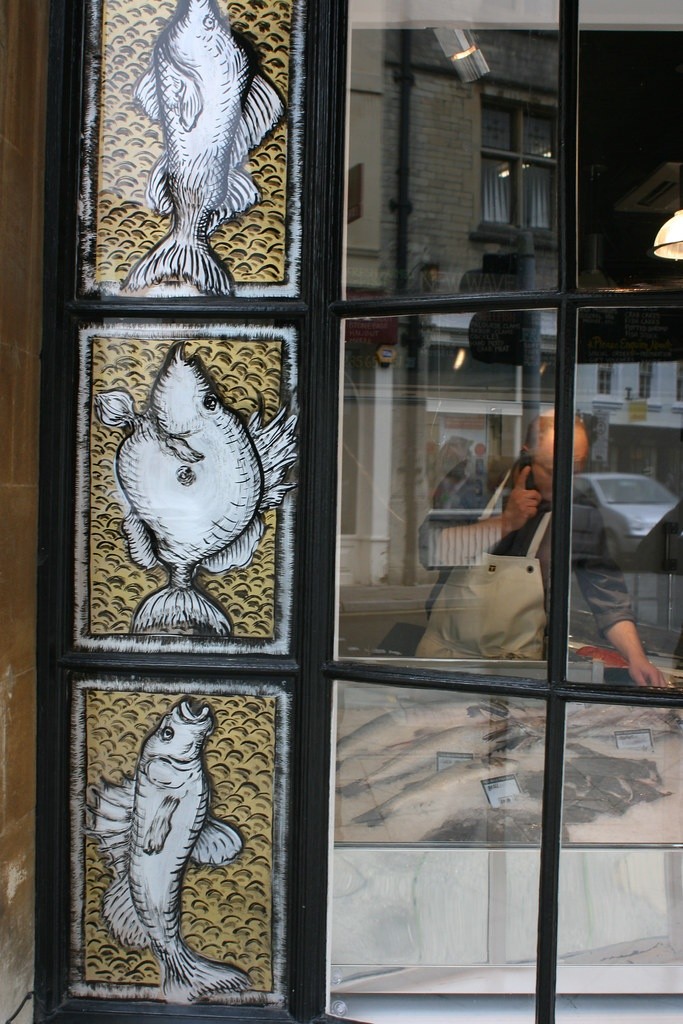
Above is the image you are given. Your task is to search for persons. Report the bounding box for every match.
[413,407,667,687]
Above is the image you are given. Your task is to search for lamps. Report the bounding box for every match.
[647,210,683,262]
[433,25,489,82]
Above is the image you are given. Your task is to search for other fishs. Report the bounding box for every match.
[335,717,546,799]
[350,737,546,827]
[336,697,526,771]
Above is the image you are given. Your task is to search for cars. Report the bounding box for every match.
[575,471,677,565]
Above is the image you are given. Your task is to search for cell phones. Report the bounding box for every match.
[520,456,535,490]
